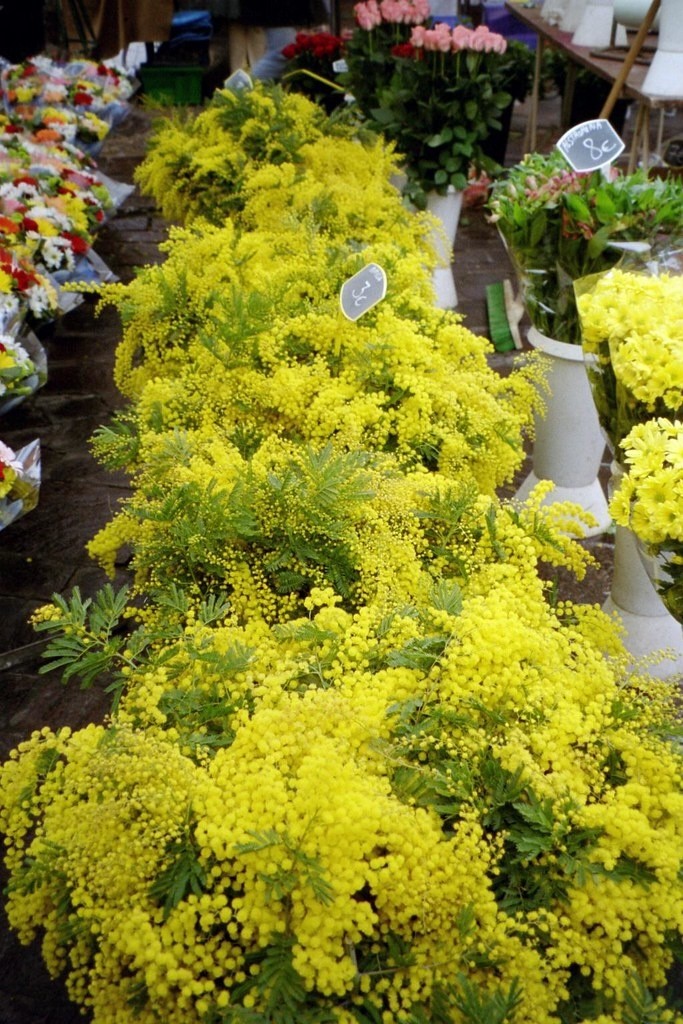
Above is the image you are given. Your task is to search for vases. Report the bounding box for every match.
[391,168,476,309]
[509,324,613,540]
[642,0,683,96]
[597,459,683,685]
[570,0,628,47]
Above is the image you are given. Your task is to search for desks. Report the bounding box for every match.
[503,0,683,158]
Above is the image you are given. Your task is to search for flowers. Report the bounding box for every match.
[481,149,683,343]
[131,78,325,224]
[0,41,161,160]
[60,210,454,381]
[0,262,58,414]
[83,419,603,618]
[86,286,555,494]
[280,0,528,211]
[0,583,683,1024]
[0,438,42,530]
[607,417,683,624]
[219,123,410,241]
[570,267,683,454]
[0,196,121,326]
[0,114,137,243]
